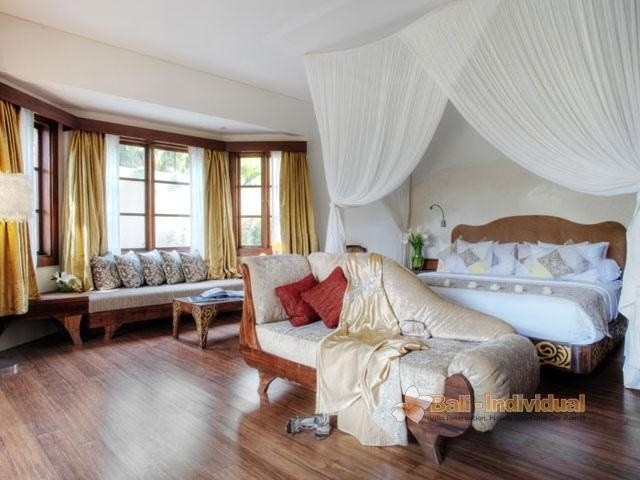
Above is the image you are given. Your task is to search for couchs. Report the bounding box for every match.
[86,249,247,341]
[240,251,541,465]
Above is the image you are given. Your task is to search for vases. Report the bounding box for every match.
[411,249,425,272]
[58,286,73,292]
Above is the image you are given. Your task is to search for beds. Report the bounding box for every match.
[416,215,630,375]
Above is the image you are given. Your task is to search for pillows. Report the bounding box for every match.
[136,249,166,286]
[114,250,144,288]
[439,234,610,282]
[275,273,321,327]
[89,251,123,291]
[158,248,186,285]
[300,266,353,329]
[178,249,207,283]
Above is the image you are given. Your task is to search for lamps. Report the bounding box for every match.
[429,203,446,228]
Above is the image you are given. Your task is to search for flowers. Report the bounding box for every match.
[53,270,84,291]
[401,226,432,251]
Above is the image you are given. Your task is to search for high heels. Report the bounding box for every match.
[285,414,331,440]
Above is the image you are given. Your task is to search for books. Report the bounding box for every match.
[188,288,243,303]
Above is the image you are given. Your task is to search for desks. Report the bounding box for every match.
[0,290,89,345]
[173,293,244,351]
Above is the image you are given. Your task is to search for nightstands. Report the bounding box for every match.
[409,258,437,274]
